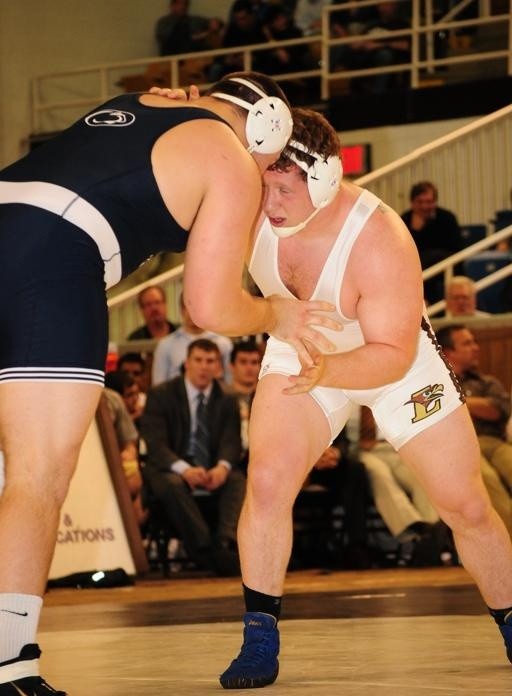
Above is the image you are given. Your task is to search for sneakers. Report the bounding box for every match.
[0,643,67,695]
[221,611,281,687]
[490,609,512,662]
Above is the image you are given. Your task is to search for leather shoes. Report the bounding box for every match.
[205,543,230,576]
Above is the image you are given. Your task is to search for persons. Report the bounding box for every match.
[0,65,346,694]
[94,1,512,578]
[145,75,512,689]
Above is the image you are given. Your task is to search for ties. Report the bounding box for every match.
[193,392,211,468]
